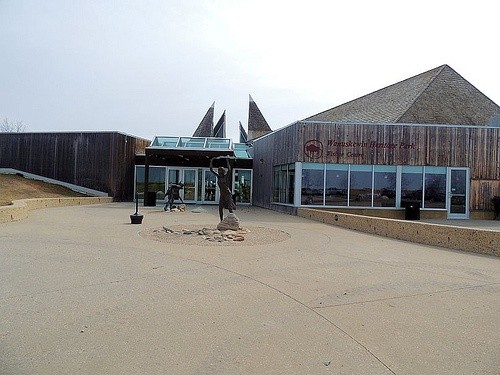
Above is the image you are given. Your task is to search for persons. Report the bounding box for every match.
[162,183,177,212]
[209,157,236,223]
[172,181,184,205]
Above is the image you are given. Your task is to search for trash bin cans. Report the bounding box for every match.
[404,199,421,221]
[492,195,500,221]
[147,191,158,207]
